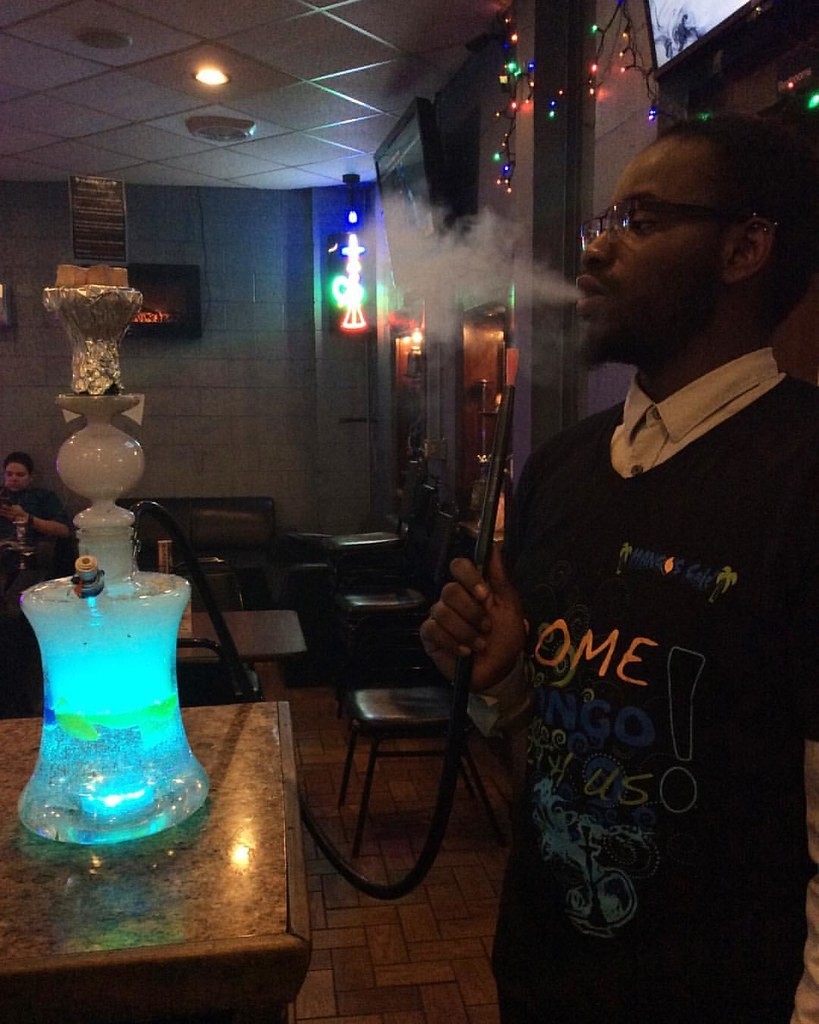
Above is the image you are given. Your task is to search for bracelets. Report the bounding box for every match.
[468,661,531,739]
[29,514,33,525]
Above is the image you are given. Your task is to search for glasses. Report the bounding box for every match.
[581,195,747,251]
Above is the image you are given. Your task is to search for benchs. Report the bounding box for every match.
[116,497,277,613]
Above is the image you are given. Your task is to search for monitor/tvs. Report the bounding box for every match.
[373,96,463,293]
[645,0,764,81]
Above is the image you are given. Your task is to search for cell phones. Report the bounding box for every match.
[0,497,13,507]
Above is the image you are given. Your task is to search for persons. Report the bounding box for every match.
[0,452,69,538]
[677,15,692,53]
[420,114,819,1023]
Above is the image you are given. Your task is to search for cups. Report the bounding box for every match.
[12,520,27,540]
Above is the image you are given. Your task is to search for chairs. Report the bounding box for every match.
[324,461,509,859]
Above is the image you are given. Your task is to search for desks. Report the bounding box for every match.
[0,701,313,1024]
[176,608,308,663]
